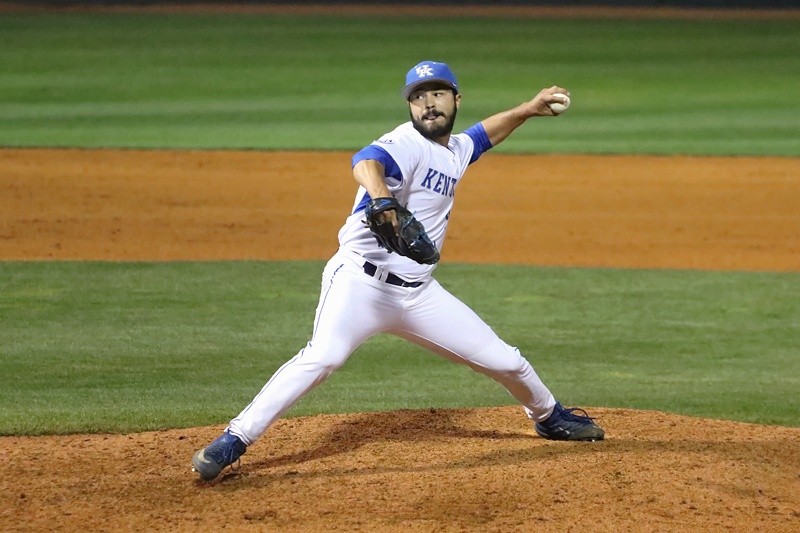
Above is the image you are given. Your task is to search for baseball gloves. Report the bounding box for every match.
[364,197,440,265]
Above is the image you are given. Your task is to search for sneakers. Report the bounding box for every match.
[192,427,247,480]
[535,402,605,441]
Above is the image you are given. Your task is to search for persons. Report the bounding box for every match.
[193,60,605,482]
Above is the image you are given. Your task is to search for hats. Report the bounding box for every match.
[404,61,459,101]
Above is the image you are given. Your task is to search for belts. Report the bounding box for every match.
[363,261,423,288]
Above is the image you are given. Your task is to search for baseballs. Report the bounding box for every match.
[548,93,570,113]
